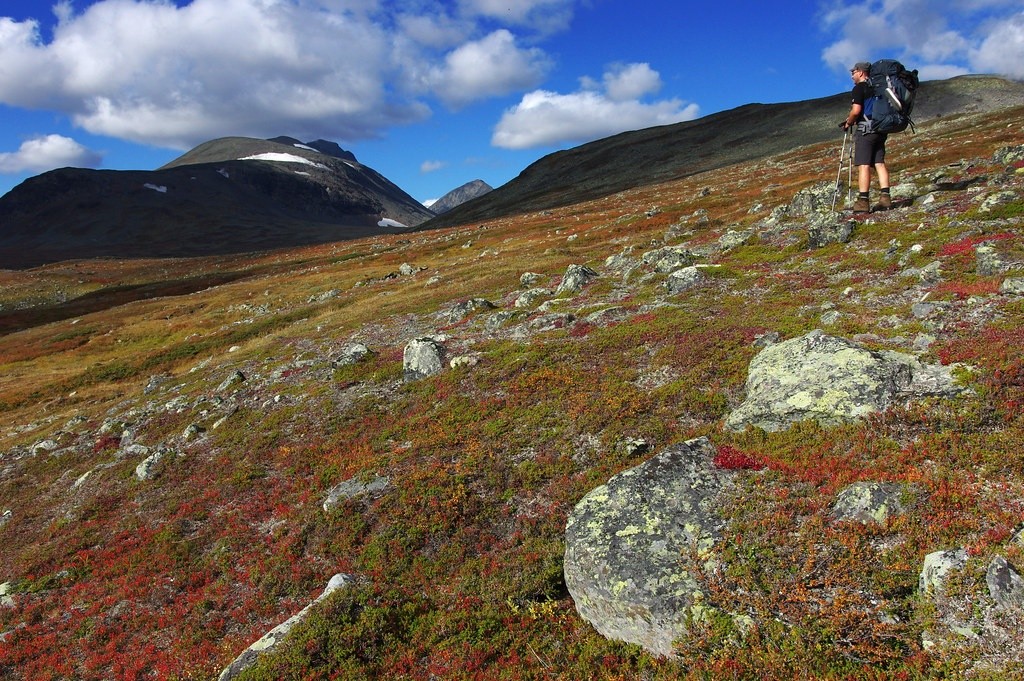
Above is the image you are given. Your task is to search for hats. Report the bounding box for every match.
[850,62,871,72]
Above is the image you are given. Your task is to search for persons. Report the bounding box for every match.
[844,62,891,212]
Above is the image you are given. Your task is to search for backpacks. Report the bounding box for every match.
[870,59,919,133]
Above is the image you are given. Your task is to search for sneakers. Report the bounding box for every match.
[872,195,892,211]
[852,199,870,214]
